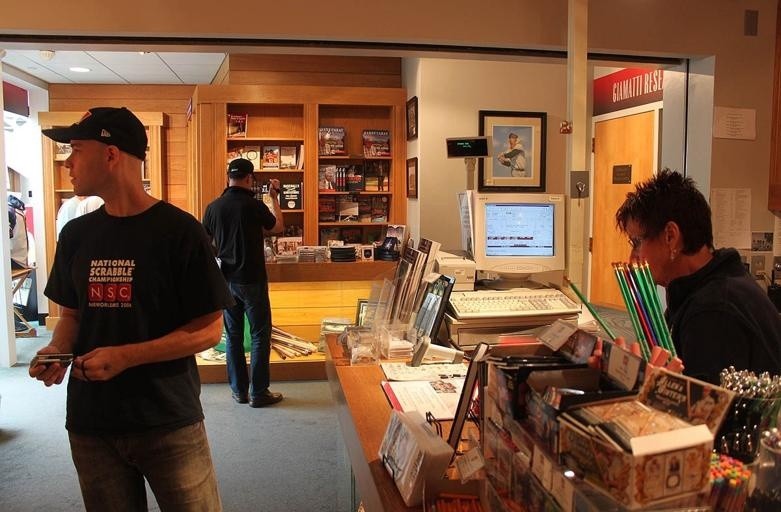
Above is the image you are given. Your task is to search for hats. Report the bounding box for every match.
[41,107,146,160]
[228,158,255,180]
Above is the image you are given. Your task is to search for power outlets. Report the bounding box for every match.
[750,255,766,281]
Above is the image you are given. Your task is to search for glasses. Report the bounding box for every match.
[628,229,657,248]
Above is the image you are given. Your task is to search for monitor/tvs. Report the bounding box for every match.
[469,193,566,286]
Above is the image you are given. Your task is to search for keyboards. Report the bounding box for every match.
[447,288,583,318]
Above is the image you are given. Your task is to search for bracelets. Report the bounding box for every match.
[78,354,100,385]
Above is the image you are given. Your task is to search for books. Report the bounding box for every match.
[227,106,394,250]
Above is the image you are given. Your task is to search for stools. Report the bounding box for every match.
[12,267,38,338]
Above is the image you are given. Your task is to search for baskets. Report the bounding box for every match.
[298,246,328,262]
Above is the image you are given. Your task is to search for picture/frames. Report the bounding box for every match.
[412,273,456,343]
[405,157,417,199]
[354,298,388,326]
[479,110,547,192]
[405,96,418,141]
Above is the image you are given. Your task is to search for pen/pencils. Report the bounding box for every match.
[718,366,781,461]
[562,260,678,368]
[705,453,752,512]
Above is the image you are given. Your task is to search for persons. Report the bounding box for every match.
[27,109,235,512]
[496,131,533,176]
[204,156,287,409]
[50,194,105,239]
[319,167,336,191]
[615,166,776,389]
[8,192,30,333]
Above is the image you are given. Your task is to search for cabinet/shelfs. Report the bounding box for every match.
[315,154,394,245]
[37,111,169,330]
[227,136,305,245]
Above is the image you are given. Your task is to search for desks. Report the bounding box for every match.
[323,331,780,512]
[265,260,400,326]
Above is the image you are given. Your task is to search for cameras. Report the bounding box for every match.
[259,177,286,196]
[32,351,74,373]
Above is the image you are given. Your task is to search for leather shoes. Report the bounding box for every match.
[231,392,249,403]
[249,393,282,407]
